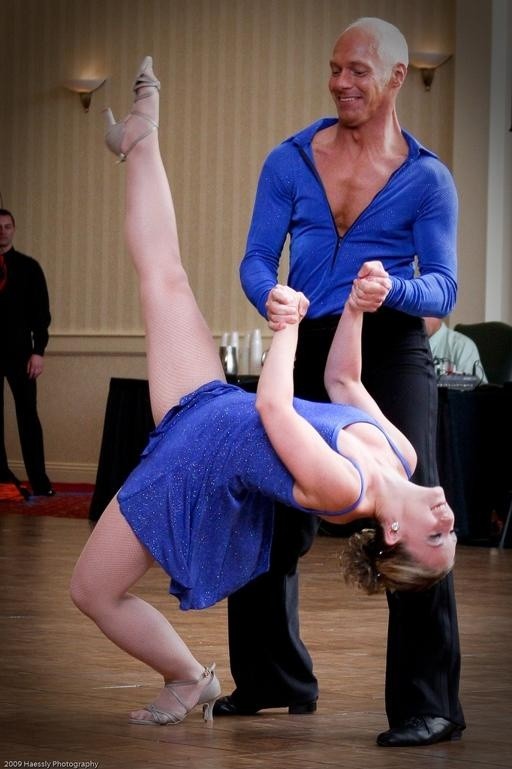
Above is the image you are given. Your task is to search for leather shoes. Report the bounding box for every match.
[35,486,55,496]
[377,715,462,746]
[203,694,318,714]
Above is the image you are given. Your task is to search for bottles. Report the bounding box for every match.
[219,329,263,376]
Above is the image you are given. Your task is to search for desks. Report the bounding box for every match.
[90,378,512,549]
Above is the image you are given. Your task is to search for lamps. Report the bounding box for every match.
[408,53,454,89]
[63,78,105,111]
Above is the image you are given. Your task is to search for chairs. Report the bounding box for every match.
[454,322,512,377]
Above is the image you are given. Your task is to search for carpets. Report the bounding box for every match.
[0,482,112,518]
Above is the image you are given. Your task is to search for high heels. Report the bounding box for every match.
[130,661,221,726]
[106,57,162,162]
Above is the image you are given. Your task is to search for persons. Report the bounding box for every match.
[422,317,488,388]
[208,16,466,747]
[0,208,59,501]
[68,55,461,730]
